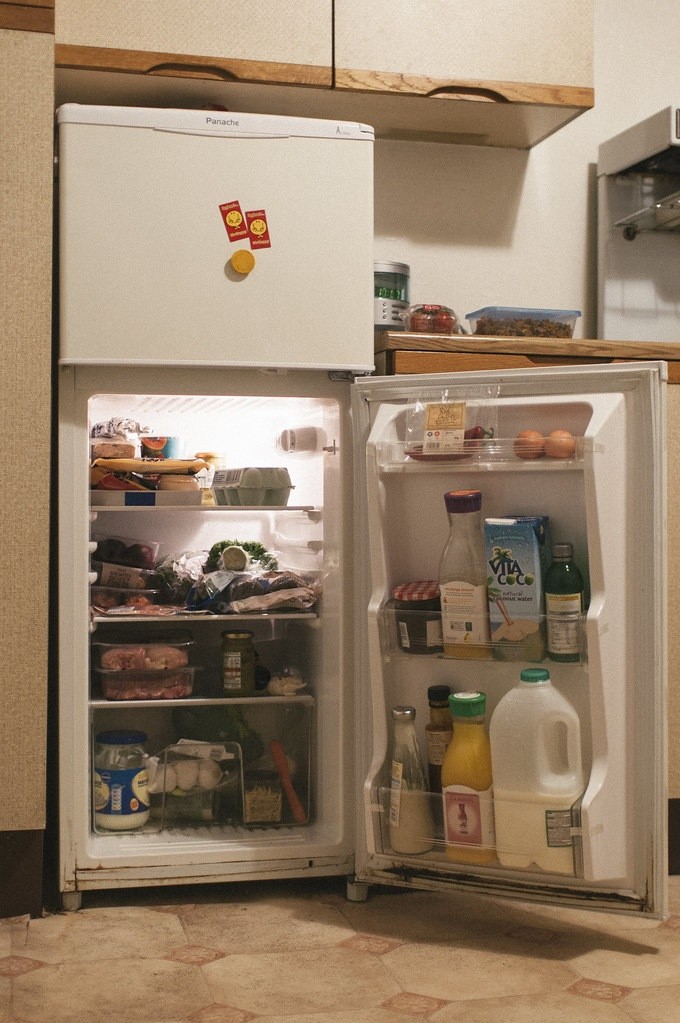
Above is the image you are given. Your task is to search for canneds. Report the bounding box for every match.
[94,729,150,829]
[222,630,255,695]
[244,770,281,823]
[392,580,444,653]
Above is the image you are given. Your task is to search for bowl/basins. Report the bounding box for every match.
[90,529,167,613]
[136,433,183,460]
[91,629,197,669]
[399,304,457,335]
[464,307,581,338]
[92,661,208,701]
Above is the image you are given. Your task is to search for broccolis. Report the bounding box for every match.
[208,540,277,574]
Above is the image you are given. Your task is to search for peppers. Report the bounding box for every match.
[405,427,496,462]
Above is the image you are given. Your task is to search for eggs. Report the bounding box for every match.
[513,431,545,459]
[544,430,575,457]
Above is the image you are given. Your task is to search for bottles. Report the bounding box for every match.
[440,690,498,865]
[388,706,437,854]
[489,668,585,875]
[543,543,586,663]
[424,685,451,851]
[438,488,492,661]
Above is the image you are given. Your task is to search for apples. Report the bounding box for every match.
[122,545,157,568]
[93,540,128,563]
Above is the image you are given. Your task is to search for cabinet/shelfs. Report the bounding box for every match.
[54,0,594,152]
[373,330,680,876]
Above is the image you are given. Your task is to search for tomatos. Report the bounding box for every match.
[434,312,453,332]
[410,314,433,332]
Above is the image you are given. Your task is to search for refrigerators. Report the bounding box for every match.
[52,104,669,928]
